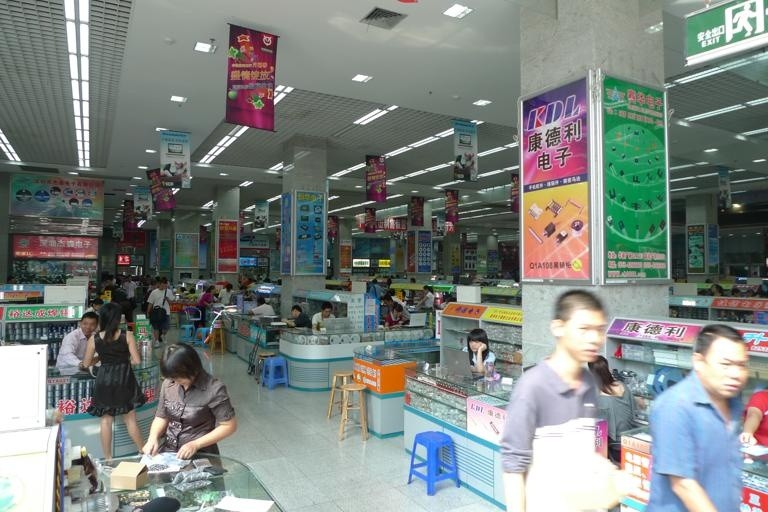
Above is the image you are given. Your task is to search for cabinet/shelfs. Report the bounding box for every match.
[3,281,90,370]
[389,282,522,312]
[49,341,163,423]
[351,344,417,440]
[68,451,287,512]
[246,281,381,333]
[1,233,100,308]
[402,301,768,512]
[204,303,291,366]
[277,327,440,393]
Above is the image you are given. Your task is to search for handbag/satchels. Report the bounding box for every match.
[151,306,166,321]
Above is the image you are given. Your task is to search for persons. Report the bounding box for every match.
[366,275,434,330]
[140,342,237,499]
[93,271,238,342]
[646,323,748,512]
[739,391,767,460]
[82,301,144,463]
[247,297,275,317]
[587,356,634,444]
[281,305,310,328]
[462,325,496,376]
[6,275,16,284]
[499,289,608,512]
[310,302,336,330]
[55,312,99,376]
[678,270,767,321]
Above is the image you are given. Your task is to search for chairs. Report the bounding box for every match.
[184,305,202,322]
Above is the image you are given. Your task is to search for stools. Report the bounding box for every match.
[338,383,367,441]
[328,369,352,421]
[180,325,195,342]
[255,352,277,383]
[264,357,290,390]
[195,327,209,348]
[407,429,461,495]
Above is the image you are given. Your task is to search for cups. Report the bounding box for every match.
[319,328,327,334]
[485,362,495,381]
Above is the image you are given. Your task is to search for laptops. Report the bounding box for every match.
[442,345,484,381]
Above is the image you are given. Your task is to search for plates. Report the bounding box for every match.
[351,334,361,343]
[340,335,350,344]
[309,335,319,345]
[330,335,340,345]
[297,335,306,344]
